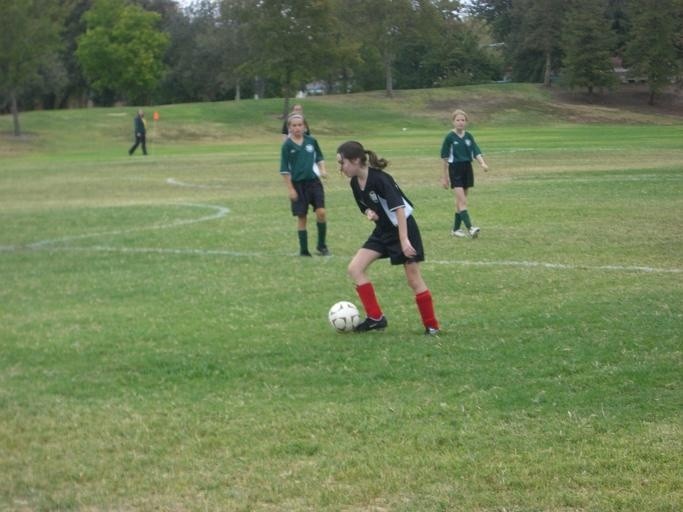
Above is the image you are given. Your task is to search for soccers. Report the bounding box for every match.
[328,300,360,333]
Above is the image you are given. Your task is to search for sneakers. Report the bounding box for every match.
[353,316,389,333]
[301,247,331,256]
[423,327,441,337]
[450,227,480,238]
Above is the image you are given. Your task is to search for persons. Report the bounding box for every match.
[440,109,489,238]
[278,112,331,258]
[281,103,310,140]
[335,140,441,336]
[128,108,149,156]
